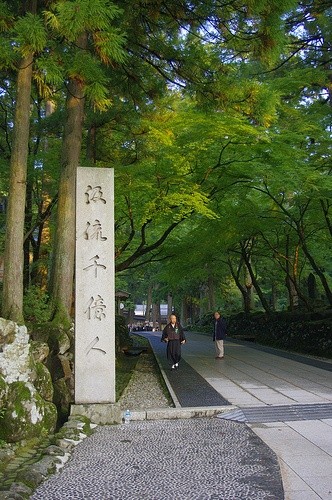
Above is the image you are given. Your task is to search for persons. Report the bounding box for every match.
[126,320,157,334]
[162,315,186,370]
[212,312,226,360]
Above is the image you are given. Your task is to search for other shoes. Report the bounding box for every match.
[217,357,224,360]
[171,367,176,370]
[175,363,179,368]
